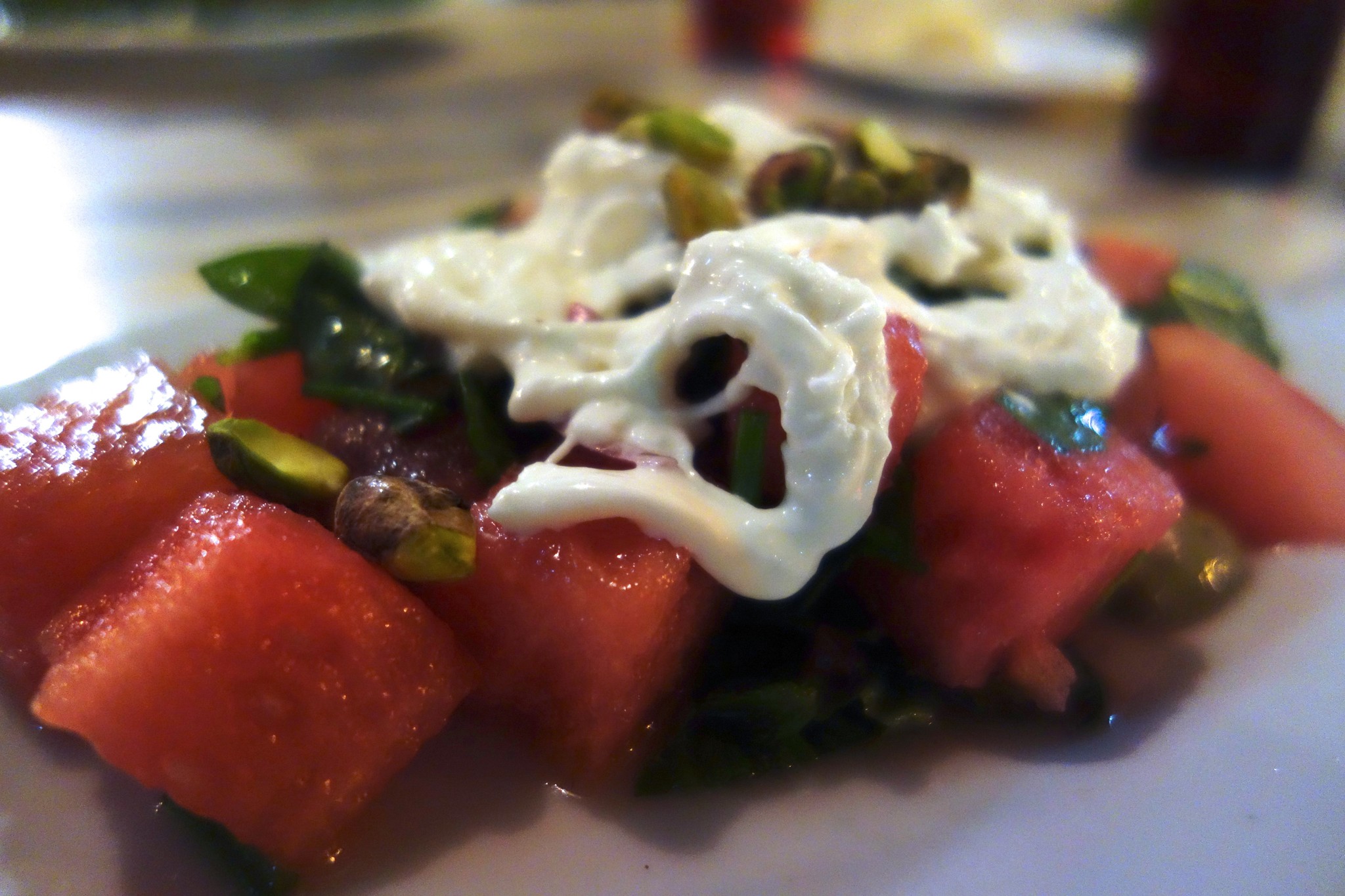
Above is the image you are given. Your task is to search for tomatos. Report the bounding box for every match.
[0,322,1345,872]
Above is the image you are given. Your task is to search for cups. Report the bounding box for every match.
[1128,0,1344,183]
[679,0,815,65]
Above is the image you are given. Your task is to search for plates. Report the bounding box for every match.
[1,1,444,58]
[798,23,1152,104]
[0,291,1344,894]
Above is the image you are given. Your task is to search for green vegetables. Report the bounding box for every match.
[191,95,1281,804]
